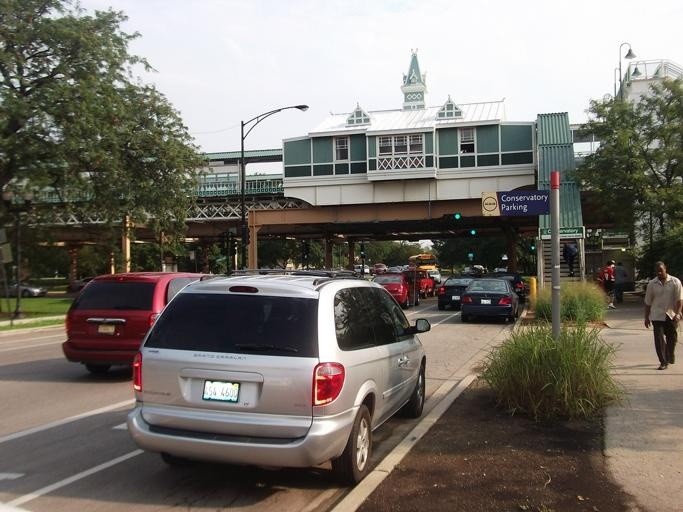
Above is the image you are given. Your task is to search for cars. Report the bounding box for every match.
[355,264,442,310]
[127,276,430,489]
[437,275,526,323]
[63,270,217,373]
[1,282,47,298]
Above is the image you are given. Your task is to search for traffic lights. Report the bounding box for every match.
[471,229,476,235]
[443,213,461,222]
[530,239,536,254]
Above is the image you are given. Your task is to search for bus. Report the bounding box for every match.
[408,252,437,270]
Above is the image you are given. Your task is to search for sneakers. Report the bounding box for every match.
[658,359,674,370]
[606,303,616,310]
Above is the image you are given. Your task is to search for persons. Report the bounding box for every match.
[611,261,630,304]
[602,260,618,310]
[597,266,606,288]
[561,242,578,278]
[642,261,683,371]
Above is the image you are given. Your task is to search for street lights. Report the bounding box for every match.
[615,42,635,98]
[241,105,309,270]
